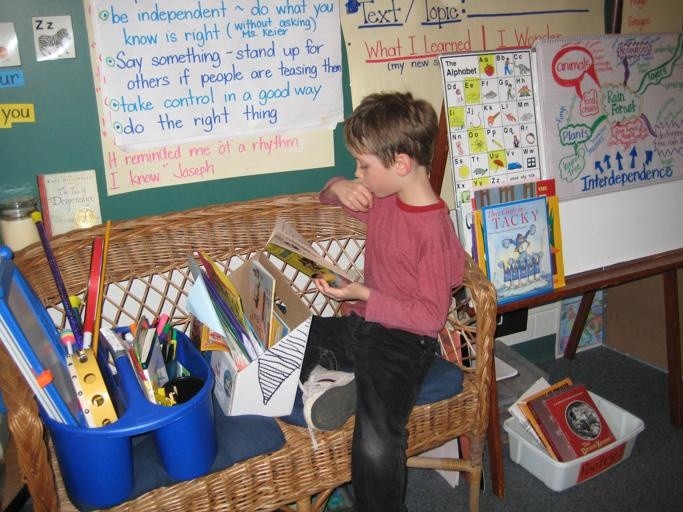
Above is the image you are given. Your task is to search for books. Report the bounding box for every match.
[36,170,103,241]
[508,376,616,462]
[265,215,355,290]
[470,179,567,306]
[186,248,290,371]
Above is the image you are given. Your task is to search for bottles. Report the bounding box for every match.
[0,195,40,252]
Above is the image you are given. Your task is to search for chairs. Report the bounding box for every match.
[0,183,497,512]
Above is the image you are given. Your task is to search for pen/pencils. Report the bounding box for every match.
[156,314,168,337]
[167,329,177,363]
[159,323,171,360]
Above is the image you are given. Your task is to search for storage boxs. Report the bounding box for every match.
[503,391,644,493]
[36,326,214,511]
[190,252,313,418]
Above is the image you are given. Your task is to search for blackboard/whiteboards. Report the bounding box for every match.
[440,46,683,308]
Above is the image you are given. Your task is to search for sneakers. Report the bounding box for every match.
[303,372,358,431]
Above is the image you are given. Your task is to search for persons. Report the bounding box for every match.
[299,91,465,512]
